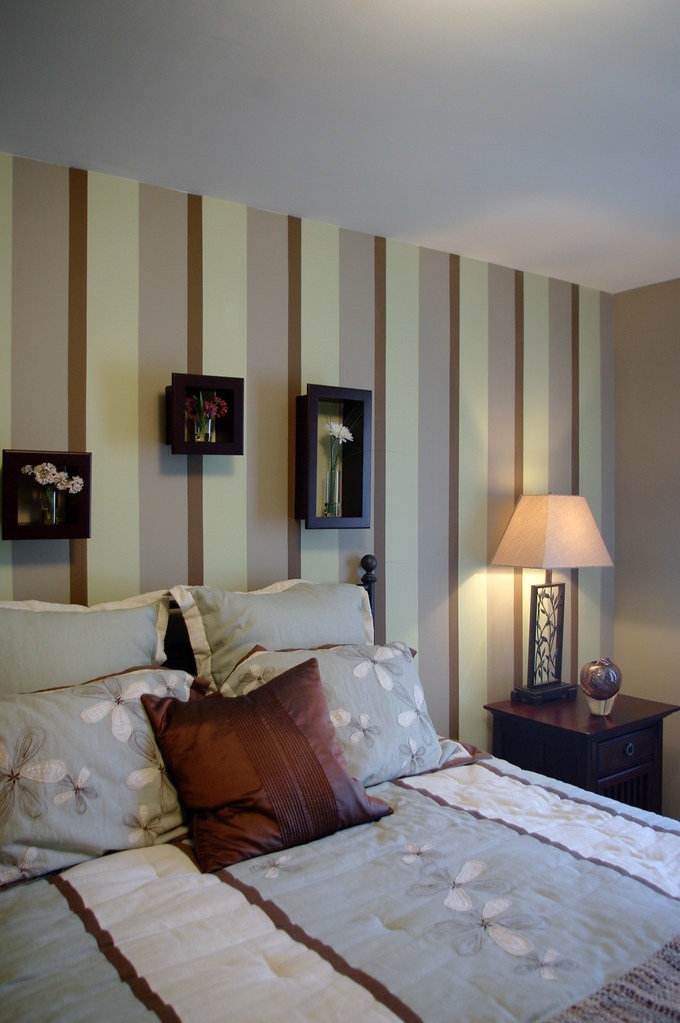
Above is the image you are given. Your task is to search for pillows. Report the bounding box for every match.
[1,587,174,695]
[168,578,374,691]
[0,666,215,891]
[220,642,493,790]
[141,658,392,874]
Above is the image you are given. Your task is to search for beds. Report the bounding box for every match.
[0,553,680,1023]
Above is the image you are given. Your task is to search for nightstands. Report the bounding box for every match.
[481,686,679,817]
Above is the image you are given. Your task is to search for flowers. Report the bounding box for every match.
[20,462,85,494]
[320,407,360,470]
[186,393,230,419]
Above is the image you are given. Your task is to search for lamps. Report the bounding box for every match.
[491,495,614,706]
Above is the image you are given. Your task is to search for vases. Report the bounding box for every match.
[322,466,342,517]
[30,488,57,522]
[186,414,215,442]
